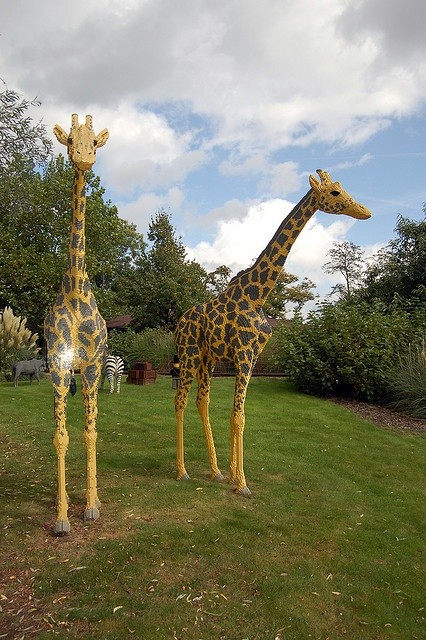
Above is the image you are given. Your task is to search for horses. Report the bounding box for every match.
[11,358,47,387]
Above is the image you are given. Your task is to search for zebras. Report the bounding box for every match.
[100,344,124,394]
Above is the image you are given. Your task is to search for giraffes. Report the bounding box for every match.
[173,169,372,499]
[42,113,107,536]
